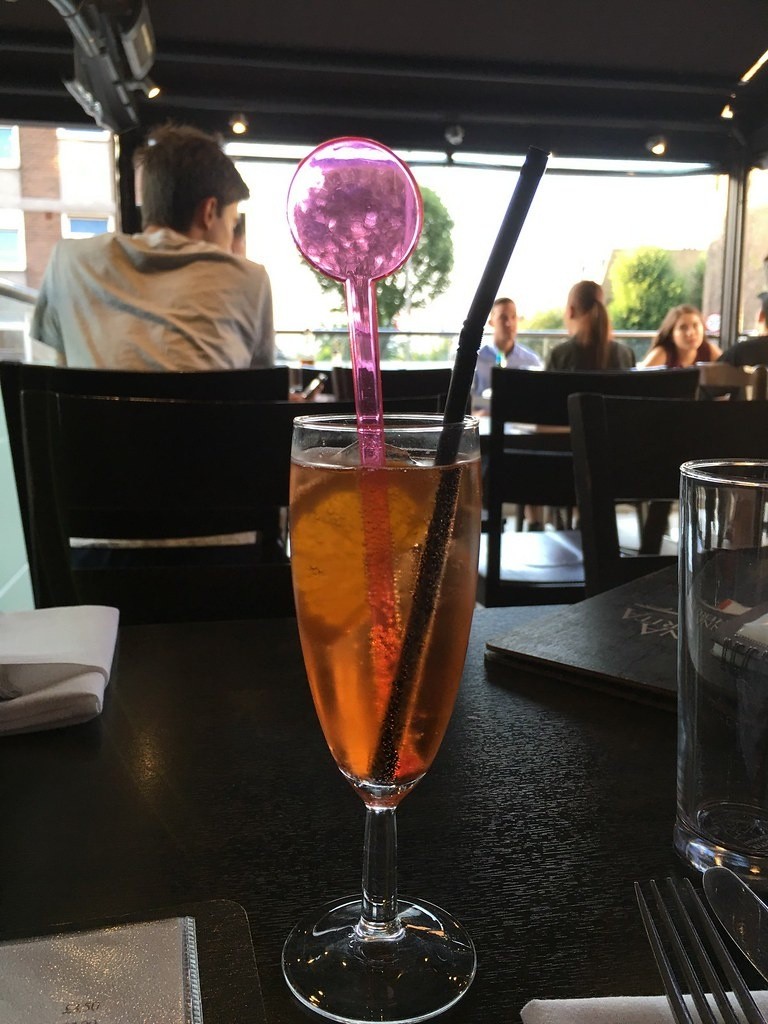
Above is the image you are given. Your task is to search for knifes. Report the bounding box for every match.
[701,860,768,985]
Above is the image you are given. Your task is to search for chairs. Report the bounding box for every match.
[0,359,291,549]
[472,366,704,611]
[16,387,479,638]
[565,391,768,598]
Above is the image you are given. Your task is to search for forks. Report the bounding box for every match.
[633,875,762,1024]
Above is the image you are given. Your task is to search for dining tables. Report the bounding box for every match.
[0,601,768,1024]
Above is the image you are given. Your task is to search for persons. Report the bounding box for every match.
[639,304,720,368]
[469,298,542,416]
[704,292,767,400]
[526,281,636,532]
[30,125,273,372]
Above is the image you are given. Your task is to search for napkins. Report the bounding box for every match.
[0,604,120,738]
[520,987,768,1024]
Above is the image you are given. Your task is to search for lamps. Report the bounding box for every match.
[446,124,464,146]
[646,134,667,155]
[228,113,249,135]
[140,75,161,98]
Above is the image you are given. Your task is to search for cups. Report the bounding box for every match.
[676,459,768,883]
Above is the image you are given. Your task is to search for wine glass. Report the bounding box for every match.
[279,413,479,1017]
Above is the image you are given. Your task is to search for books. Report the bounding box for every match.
[484,549,768,724]
